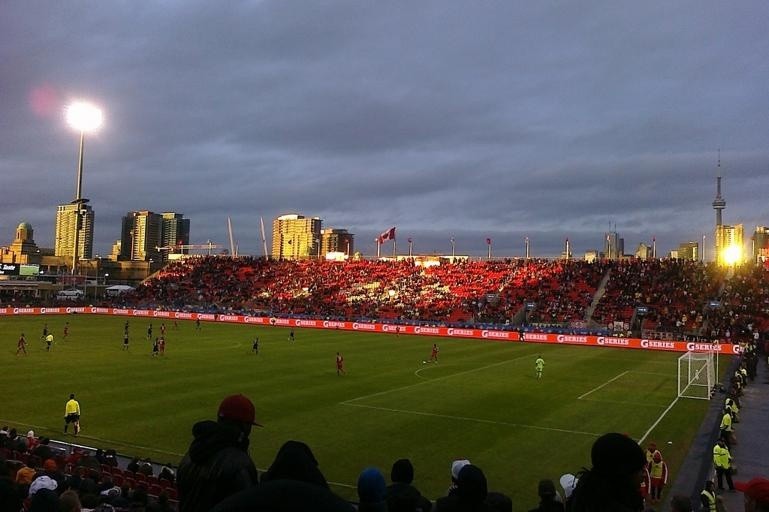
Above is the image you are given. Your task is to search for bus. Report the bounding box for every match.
[103,285,136,301]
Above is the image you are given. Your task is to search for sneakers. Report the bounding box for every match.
[650,497,662,505]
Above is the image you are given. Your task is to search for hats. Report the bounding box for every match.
[390,458,415,485]
[26,429,35,439]
[733,476,769,503]
[538,479,557,498]
[451,459,471,480]
[356,468,385,500]
[458,464,489,500]
[44,458,57,473]
[591,432,648,476]
[559,473,580,498]
[15,467,36,485]
[217,393,264,429]
[29,474,59,499]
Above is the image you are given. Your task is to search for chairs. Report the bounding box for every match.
[2,438,186,511]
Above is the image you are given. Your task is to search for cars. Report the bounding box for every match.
[57,287,83,297]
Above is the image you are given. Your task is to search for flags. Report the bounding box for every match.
[378,227,395,245]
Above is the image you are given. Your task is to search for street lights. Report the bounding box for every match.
[62,98,106,275]
